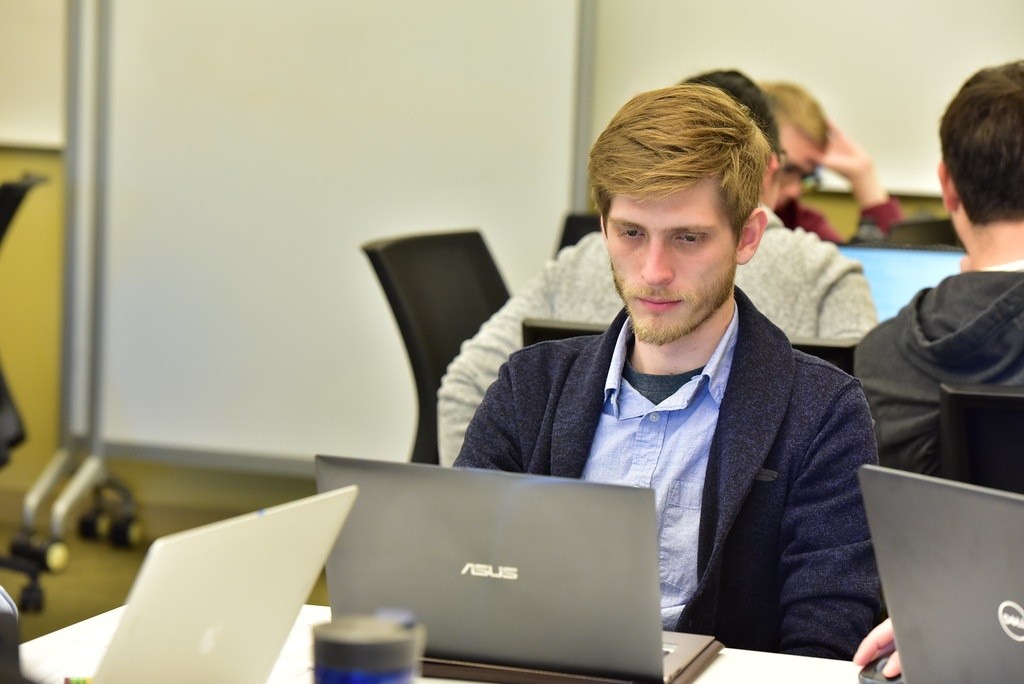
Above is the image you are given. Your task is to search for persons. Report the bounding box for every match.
[436,56,1023,680]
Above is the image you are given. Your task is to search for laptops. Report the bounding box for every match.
[311,454,725,684]
[91,485,357,684]
[858,463,1024,684]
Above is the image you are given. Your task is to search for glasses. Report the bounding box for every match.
[779,164,819,193]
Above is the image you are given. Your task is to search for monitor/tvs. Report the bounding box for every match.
[826,243,968,324]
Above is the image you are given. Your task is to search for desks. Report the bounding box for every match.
[16,596,866,684]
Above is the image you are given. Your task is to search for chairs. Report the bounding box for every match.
[360,230,513,466]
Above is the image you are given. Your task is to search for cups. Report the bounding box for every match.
[314,615,426,684]
